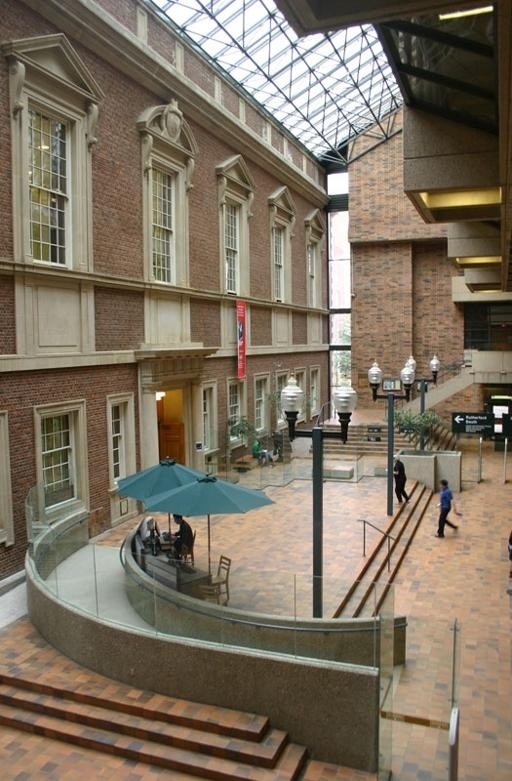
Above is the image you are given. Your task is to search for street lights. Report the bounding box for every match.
[280,373,357,619]
[406,355,440,451]
[369,359,415,516]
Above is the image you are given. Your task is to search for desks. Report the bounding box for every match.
[134,529,212,601]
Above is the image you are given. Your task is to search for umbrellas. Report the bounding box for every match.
[142,472,277,575]
[116,453,203,537]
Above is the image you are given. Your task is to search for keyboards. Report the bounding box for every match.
[163,535,169,541]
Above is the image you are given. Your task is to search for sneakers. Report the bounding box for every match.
[435,533,445,537]
[395,497,411,505]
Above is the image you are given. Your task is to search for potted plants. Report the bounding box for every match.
[229,415,258,472]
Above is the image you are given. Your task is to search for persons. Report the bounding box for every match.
[162,513,193,555]
[433,480,453,538]
[392,456,411,506]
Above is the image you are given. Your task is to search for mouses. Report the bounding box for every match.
[162,532,166,535]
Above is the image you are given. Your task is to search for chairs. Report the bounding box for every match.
[197,554,231,606]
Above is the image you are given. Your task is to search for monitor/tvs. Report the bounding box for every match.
[155,521,160,537]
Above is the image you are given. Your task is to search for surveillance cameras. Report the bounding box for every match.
[351,293,355,299]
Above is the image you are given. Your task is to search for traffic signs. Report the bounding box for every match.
[452,413,494,434]
